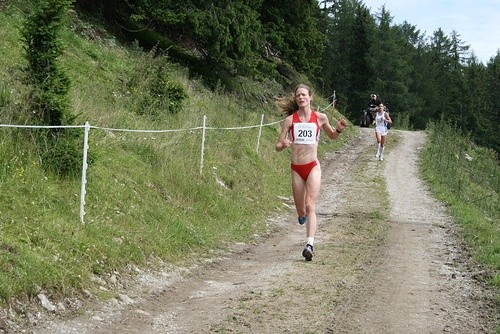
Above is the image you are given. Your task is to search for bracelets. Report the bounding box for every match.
[336,129,341,133]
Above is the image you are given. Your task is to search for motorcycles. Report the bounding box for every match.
[359,103,391,129]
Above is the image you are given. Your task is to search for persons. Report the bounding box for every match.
[274,84,348,262]
[368,94,382,108]
[372,104,392,162]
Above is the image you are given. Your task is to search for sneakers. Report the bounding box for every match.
[302,244,313,261]
[298,214,307,225]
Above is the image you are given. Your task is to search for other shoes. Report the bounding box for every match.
[375,154,380,158]
[380,157,384,162]
